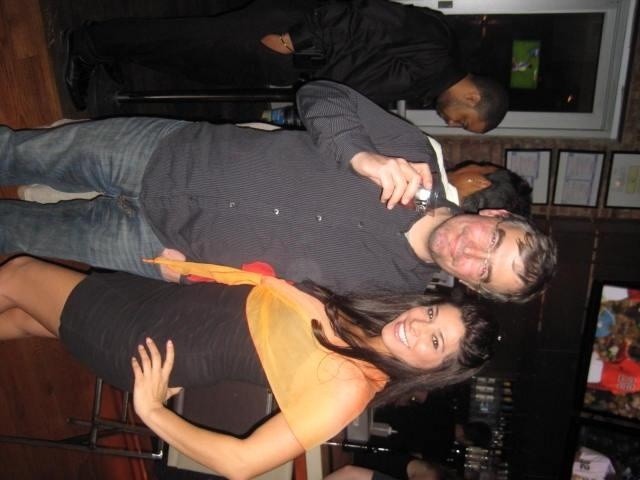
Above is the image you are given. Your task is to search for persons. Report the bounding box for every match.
[583,285,640,418]
[570,446,632,480]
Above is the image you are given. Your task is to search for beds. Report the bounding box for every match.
[421,357,527,479]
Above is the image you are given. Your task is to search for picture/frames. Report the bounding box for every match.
[397,0,640,212]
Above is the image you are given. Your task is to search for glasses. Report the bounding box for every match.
[475,220,504,293]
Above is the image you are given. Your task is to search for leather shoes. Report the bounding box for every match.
[55,16,121,113]
